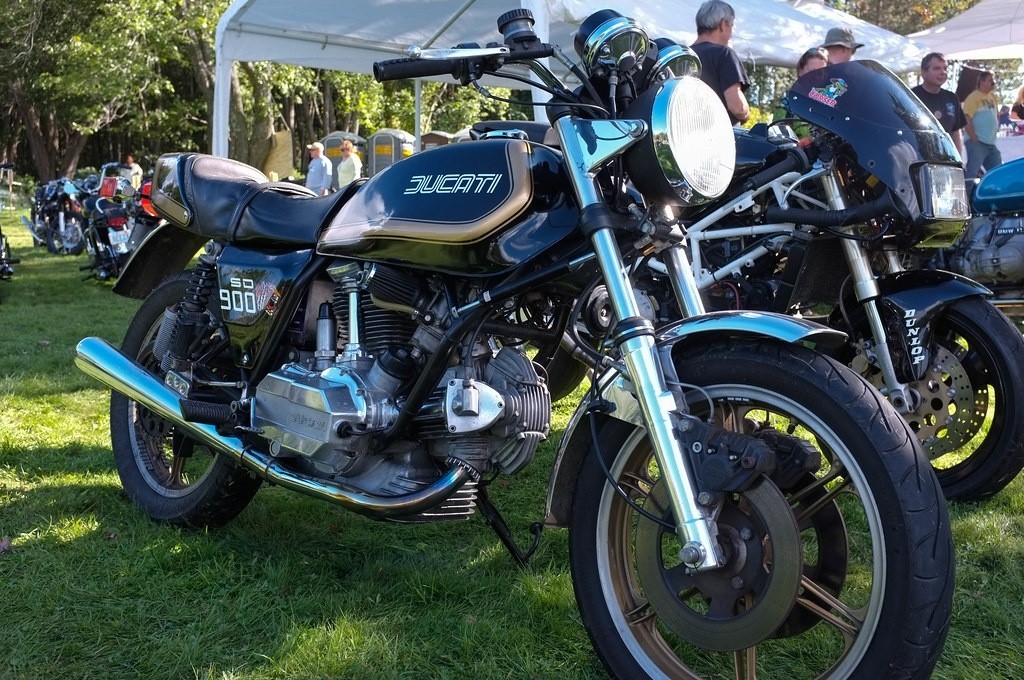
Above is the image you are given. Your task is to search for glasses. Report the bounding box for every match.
[341,149,349,152]
[808,47,827,56]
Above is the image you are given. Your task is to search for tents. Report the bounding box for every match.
[904,0,1024,60]
[212,0,923,159]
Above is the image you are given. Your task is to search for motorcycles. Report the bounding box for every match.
[0,0,1024,511]
[72,7,956,680]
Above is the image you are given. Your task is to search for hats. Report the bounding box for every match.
[307,142,324,150]
[821,27,865,48]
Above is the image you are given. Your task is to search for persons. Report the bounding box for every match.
[798,28,864,76]
[121,156,143,191]
[306,142,332,197]
[689,0,751,120]
[1000,86,1024,137]
[910,53,967,156]
[337,140,362,190]
[960,69,1002,176]
